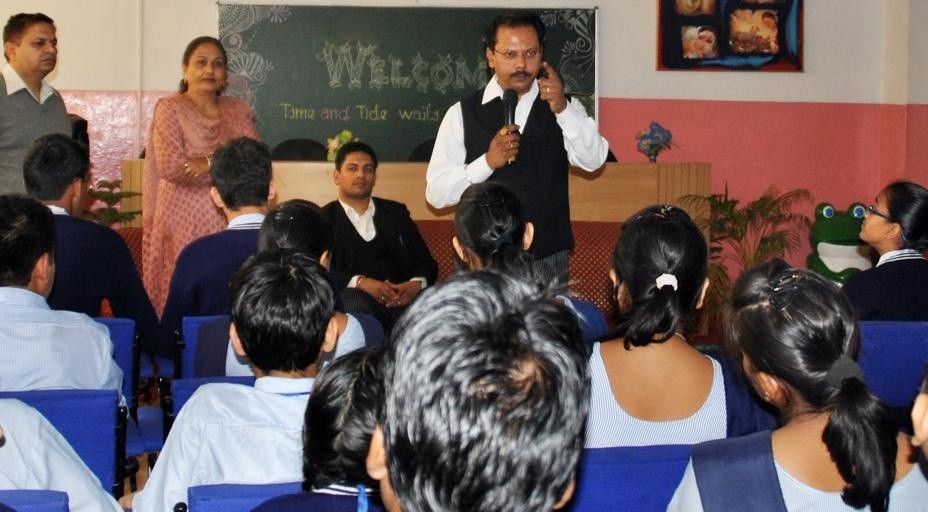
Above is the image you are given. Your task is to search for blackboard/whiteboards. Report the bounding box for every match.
[218,1,601,164]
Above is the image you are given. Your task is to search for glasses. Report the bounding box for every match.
[491,48,541,60]
[622,205,689,230]
[867,205,907,241]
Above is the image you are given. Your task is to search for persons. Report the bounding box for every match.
[1,10,75,198]
[118,246,338,512]
[423,9,611,300]
[1,396,125,511]
[317,140,438,325]
[1,190,141,417]
[139,34,261,318]
[582,201,779,450]
[243,341,387,511]
[362,265,595,511]
[908,371,928,460]
[192,198,385,377]
[760,11,780,54]
[693,28,720,58]
[838,177,928,321]
[160,134,273,339]
[22,133,159,339]
[449,181,609,353]
[663,254,928,510]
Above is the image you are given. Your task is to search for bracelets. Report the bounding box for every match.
[357,277,364,288]
[204,154,211,171]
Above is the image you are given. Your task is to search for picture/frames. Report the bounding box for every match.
[655,0,805,74]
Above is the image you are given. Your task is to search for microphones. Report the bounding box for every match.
[502,88,520,167]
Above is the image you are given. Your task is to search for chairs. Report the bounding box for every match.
[271,138,328,161]
[90,317,135,408]
[0,490,69,512]
[850,319,926,411]
[176,315,230,377]
[0,390,144,503]
[562,445,692,511]
[408,138,434,162]
[138,375,256,471]
[174,482,303,512]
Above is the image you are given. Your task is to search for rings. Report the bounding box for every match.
[381,295,384,301]
[499,125,508,137]
[186,167,191,172]
[194,174,198,177]
[546,87,550,93]
[183,161,189,169]
[511,143,514,149]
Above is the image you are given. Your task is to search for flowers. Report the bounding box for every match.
[326,128,362,163]
[635,121,677,156]
